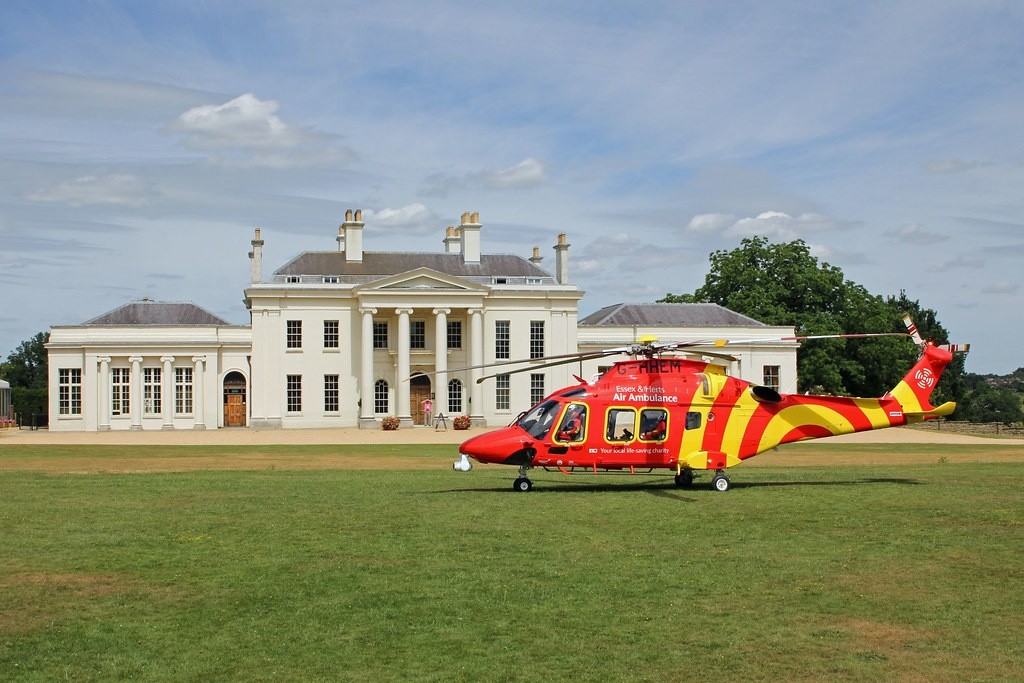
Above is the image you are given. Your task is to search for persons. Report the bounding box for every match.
[538,404,583,442]
[421,399,434,426]
[640,411,665,440]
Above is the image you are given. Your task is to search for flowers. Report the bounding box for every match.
[381,415,400,430]
[0,417,15,423]
[453,416,471,429]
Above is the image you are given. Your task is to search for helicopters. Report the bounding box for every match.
[402,312,971,492]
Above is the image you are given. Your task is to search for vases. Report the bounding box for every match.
[0,423,16,428]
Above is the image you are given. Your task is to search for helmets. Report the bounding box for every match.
[573,406,583,414]
[654,411,666,422]
